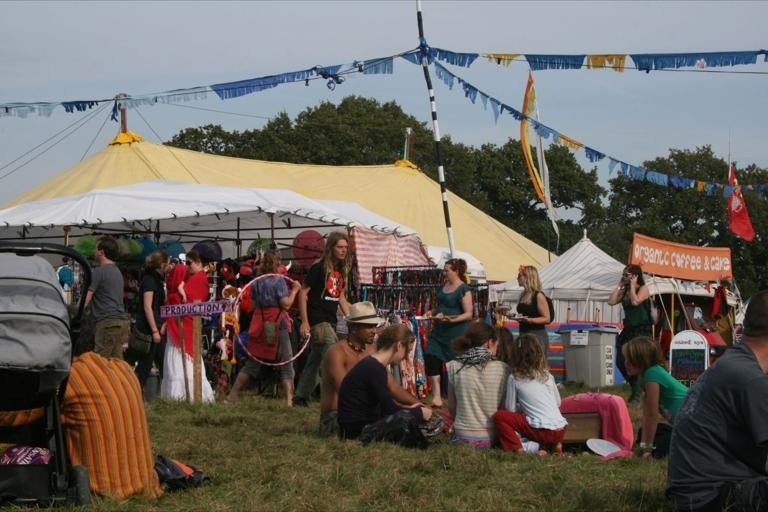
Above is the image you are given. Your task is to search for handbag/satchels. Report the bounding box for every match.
[416,408,455,444]
[154,454,212,493]
[643,297,659,325]
[130,325,152,354]
[533,290,554,324]
[263,322,274,346]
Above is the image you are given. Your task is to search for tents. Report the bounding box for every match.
[492,237,640,324]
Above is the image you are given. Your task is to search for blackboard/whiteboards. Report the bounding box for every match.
[671,349,705,381]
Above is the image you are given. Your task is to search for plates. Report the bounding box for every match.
[586,437,621,457]
[507,315,529,321]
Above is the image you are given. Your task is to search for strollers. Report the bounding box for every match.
[0,239,93,506]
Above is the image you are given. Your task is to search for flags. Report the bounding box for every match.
[727,164,758,243]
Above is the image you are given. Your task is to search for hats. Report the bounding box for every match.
[343,301,386,324]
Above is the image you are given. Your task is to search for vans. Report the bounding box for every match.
[665,304,726,367]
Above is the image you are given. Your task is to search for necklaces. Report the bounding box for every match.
[347,337,366,352]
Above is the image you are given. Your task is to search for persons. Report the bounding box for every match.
[510,265,550,354]
[337,324,433,444]
[319,301,384,437]
[2,314,162,502]
[443,323,567,455]
[424,259,473,408]
[56,231,356,407]
[667,290,768,512]
[608,264,653,400]
[621,336,688,458]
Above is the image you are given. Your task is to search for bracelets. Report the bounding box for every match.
[639,442,653,448]
